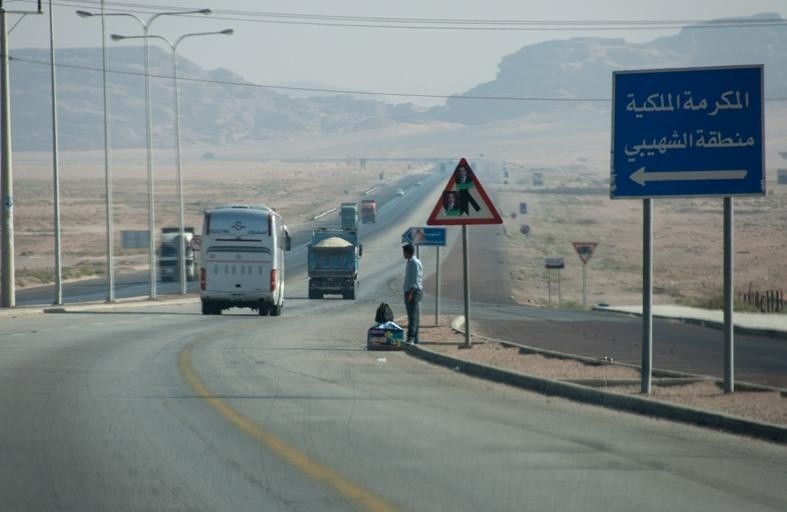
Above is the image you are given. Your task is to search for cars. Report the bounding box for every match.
[394,188,405,197]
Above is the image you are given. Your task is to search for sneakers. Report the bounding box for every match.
[405,340,414,344]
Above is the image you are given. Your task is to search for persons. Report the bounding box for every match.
[401,242,423,345]
[443,193,459,210]
[455,166,471,184]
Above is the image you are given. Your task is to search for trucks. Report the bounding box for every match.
[307,226,362,301]
[340,203,359,230]
[361,199,377,223]
[160,226,195,283]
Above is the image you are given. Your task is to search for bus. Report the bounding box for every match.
[199,204,293,318]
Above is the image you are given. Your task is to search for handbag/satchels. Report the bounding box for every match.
[376,303,393,323]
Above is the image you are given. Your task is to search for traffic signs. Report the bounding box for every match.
[402,227,446,246]
[610,64,767,199]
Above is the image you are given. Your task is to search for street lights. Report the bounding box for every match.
[111,27,234,294]
[77,7,212,304]
